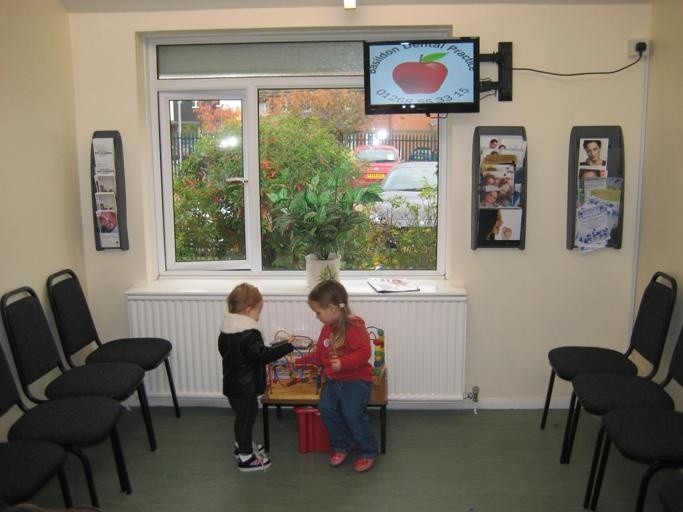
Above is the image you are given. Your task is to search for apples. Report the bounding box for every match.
[390,52,450,94]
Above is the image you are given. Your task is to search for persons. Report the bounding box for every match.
[577,169,606,180]
[479,134,526,242]
[216,282,296,472]
[289,281,376,472]
[579,137,607,167]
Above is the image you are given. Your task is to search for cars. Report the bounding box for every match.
[409,147,438,161]
[344,145,404,189]
[369,161,438,229]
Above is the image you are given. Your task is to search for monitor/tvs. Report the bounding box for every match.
[363,36,479,114]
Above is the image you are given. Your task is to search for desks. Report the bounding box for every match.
[264,377,387,459]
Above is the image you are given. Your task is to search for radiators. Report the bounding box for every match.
[127,287,476,410]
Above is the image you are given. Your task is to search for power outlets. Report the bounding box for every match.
[628,39,653,57]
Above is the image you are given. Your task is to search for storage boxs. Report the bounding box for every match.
[293,408,338,453]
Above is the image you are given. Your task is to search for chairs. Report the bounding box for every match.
[44,267,182,449]
[588,399,683,512]
[1,440,86,510]
[1,348,134,503]
[562,325,683,504]
[2,283,154,494]
[542,272,676,427]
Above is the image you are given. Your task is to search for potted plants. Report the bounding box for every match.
[266,167,386,287]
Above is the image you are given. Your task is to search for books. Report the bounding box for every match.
[92,137,120,249]
[367,277,420,293]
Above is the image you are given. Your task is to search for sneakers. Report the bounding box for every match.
[238,455,270,471]
[330,451,348,466]
[234,442,264,458]
[353,456,375,471]
